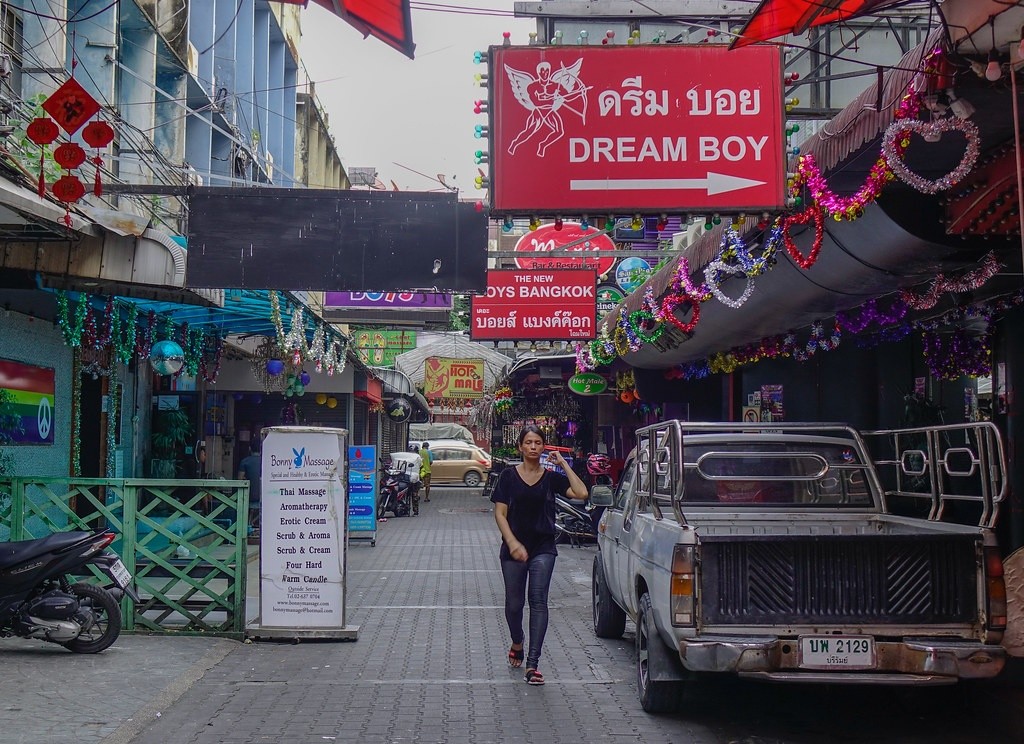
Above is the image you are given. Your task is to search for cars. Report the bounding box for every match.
[409,439,492,487]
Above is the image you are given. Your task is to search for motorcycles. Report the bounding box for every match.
[552,473,619,549]
[376,456,414,520]
[0,527,140,656]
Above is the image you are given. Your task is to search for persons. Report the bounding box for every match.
[408,444,423,517]
[199,449,213,517]
[489,425,589,685]
[416,442,433,502]
[236,438,262,529]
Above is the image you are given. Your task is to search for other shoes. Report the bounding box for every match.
[424,497,430,502]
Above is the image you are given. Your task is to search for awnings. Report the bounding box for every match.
[395,333,515,414]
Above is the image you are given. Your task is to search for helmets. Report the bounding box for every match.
[409,445,419,450]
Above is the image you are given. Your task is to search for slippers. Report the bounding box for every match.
[509,634,525,668]
[523,670,545,685]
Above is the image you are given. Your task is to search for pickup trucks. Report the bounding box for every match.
[591,419,1008,716]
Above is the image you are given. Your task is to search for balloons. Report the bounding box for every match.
[315,393,327,404]
[267,358,284,376]
[286,370,310,396]
[327,398,337,408]
[150,340,185,375]
